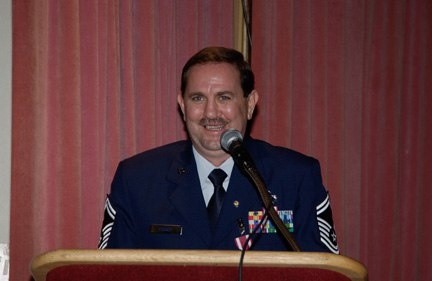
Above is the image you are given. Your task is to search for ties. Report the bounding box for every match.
[207,169,228,235]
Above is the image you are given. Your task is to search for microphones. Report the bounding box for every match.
[221,128,254,171]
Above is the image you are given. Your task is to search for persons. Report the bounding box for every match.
[98,46,339,254]
